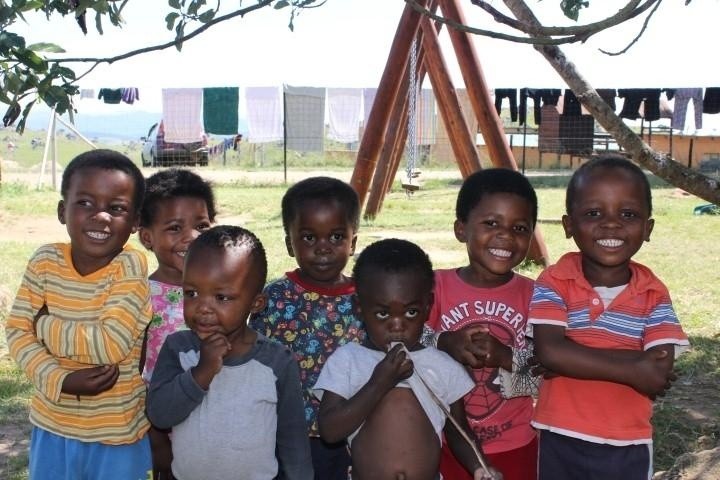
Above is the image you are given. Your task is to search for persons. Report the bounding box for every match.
[247,177,367,480]
[146,225,315,480]
[138,169,217,479]
[311,238,503,479]
[7,149,153,479]
[425,167,540,479]
[525,157,688,479]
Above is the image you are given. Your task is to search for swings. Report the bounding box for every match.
[399,41,421,196]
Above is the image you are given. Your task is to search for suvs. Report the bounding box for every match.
[138,119,209,167]
